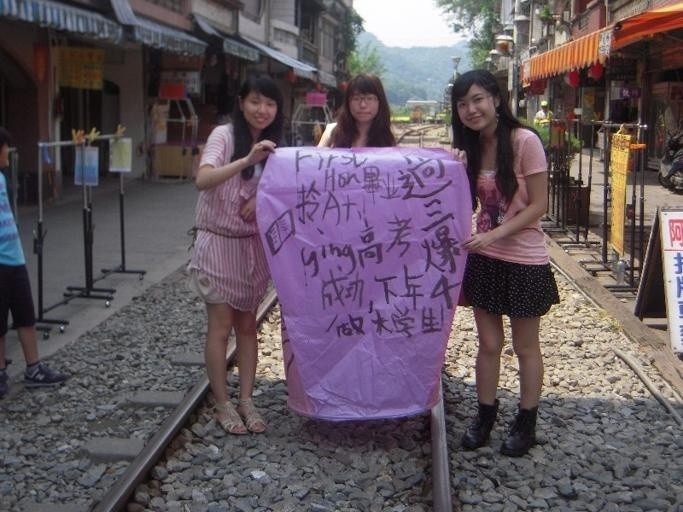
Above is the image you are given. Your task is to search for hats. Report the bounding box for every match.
[541,100,550,107]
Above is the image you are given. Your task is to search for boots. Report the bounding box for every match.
[501,404,538,456]
[462,399,499,448]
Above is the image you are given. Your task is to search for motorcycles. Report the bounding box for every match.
[657,130,683,194]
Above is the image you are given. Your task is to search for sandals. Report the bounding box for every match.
[237,400,266,433]
[212,403,247,435]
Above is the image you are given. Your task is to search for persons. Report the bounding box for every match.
[316,72,396,150]
[181,72,283,435]
[0,139,73,401]
[533,100,555,120]
[445,70,561,455]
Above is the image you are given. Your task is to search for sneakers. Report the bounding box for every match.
[0,369,8,399]
[24,363,66,387]
